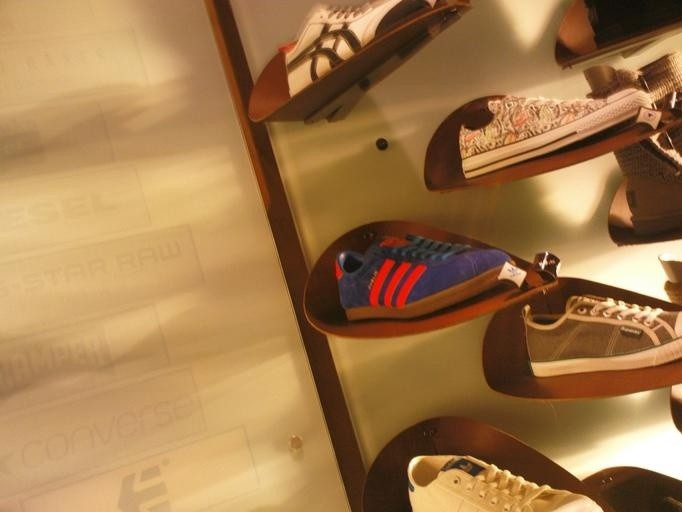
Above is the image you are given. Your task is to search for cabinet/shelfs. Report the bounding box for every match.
[206,0,682,511]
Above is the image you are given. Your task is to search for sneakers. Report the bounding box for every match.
[279,0,434,99]
[334,230,518,323]
[457,87,653,181]
[522,294,682,379]
[407,449,604,512]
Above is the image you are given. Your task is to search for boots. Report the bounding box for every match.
[579,53,682,237]
